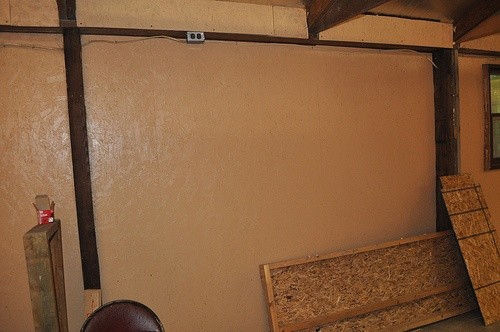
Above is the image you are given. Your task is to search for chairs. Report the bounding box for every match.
[79,298,166,332]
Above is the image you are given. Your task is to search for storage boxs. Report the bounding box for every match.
[31,195,55,226]
